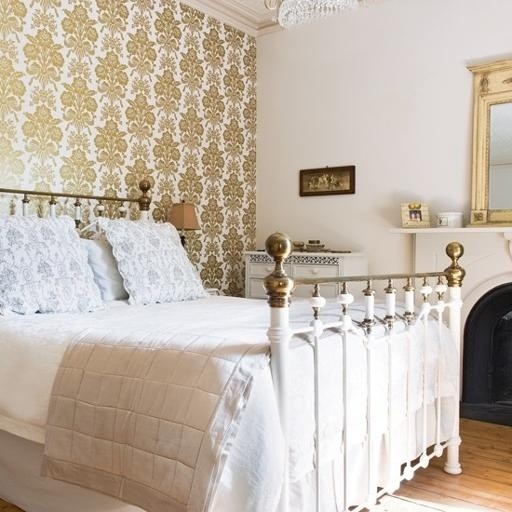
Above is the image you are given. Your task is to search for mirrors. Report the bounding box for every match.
[466,58,512,229]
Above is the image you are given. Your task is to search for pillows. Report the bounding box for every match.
[0,218,208,317]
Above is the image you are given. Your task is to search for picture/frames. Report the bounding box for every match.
[400,203,432,229]
[298,165,356,196]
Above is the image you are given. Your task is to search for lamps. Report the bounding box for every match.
[167,198,200,248]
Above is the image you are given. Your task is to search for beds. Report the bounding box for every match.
[0,180,468,511]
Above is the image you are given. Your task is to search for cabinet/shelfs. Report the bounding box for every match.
[242,251,368,301]
